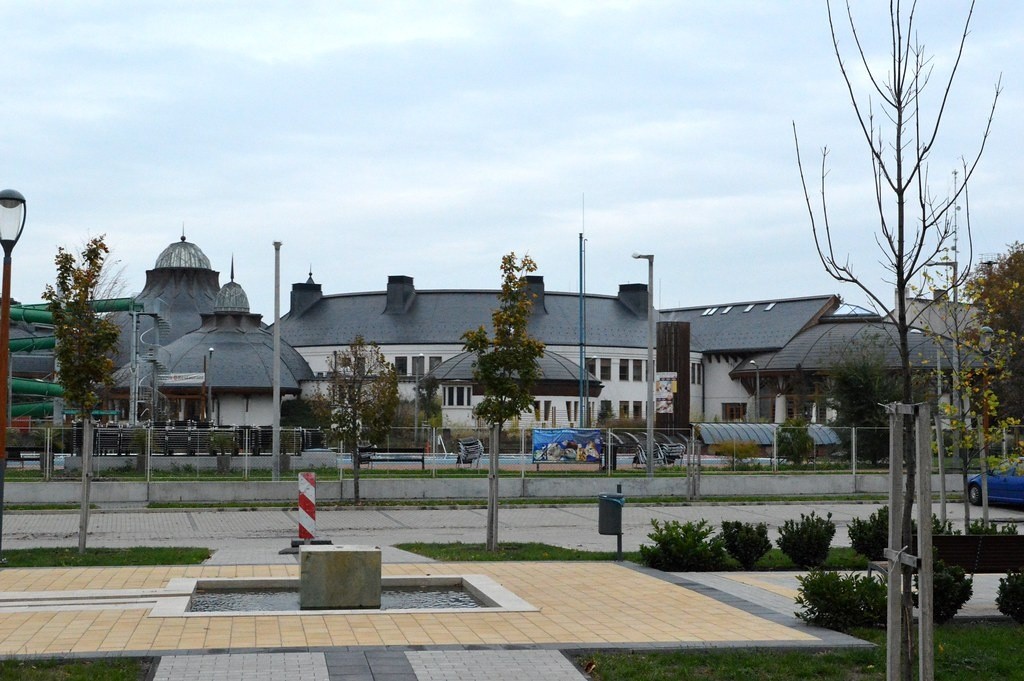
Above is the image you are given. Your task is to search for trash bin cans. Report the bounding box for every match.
[598,493,625,536]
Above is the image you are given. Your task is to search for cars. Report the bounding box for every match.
[967,457,1024,507]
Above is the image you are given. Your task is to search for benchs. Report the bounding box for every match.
[356,447,425,470]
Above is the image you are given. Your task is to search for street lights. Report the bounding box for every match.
[909,329,943,420]
[632,250,655,476]
[750,359,759,421]
[925,259,962,469]
[0,188,27,562]
[207,347,215,419]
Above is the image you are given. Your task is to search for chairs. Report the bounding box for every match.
[633,440,684,468]
[456,438,484,469]
